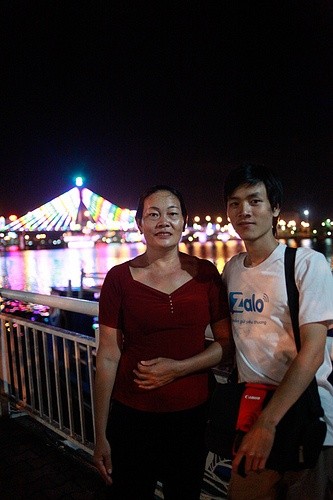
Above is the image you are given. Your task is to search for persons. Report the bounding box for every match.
[91,184,237,500]
[220,165,333,500]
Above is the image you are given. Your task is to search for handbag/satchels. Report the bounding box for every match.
[218,377,326,471]
